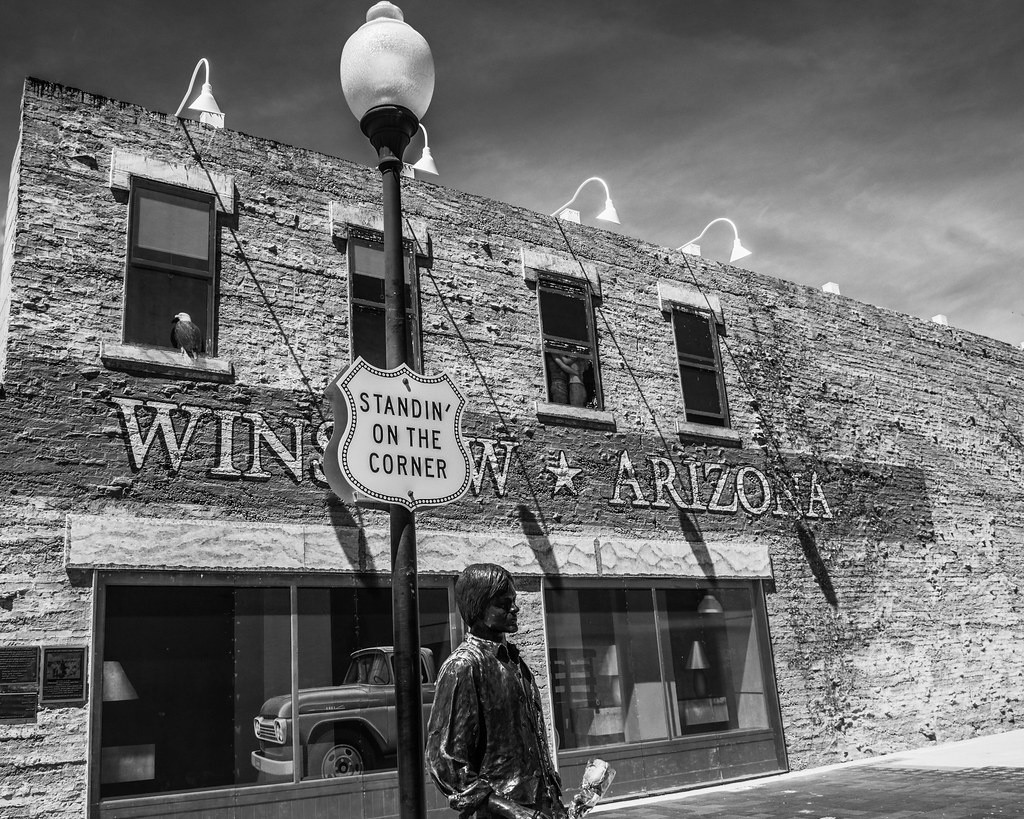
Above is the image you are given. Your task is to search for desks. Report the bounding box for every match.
[677,696,730,735]
[570,706,625,746]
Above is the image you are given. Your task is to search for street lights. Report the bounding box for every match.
[338,1,434,818]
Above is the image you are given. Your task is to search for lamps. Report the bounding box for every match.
[698,595,723,613]
[173,57,222,116]
[685,640,712,697]
[598,644,622,707]
[551,177,620,225]
[375,123,439,175]
[102,661,139,702]
[676,217,752,262]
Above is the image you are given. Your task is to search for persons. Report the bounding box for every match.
[548,352,589,407]
[425,563,569,819]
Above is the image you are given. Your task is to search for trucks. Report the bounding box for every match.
[251,644,441,782]
